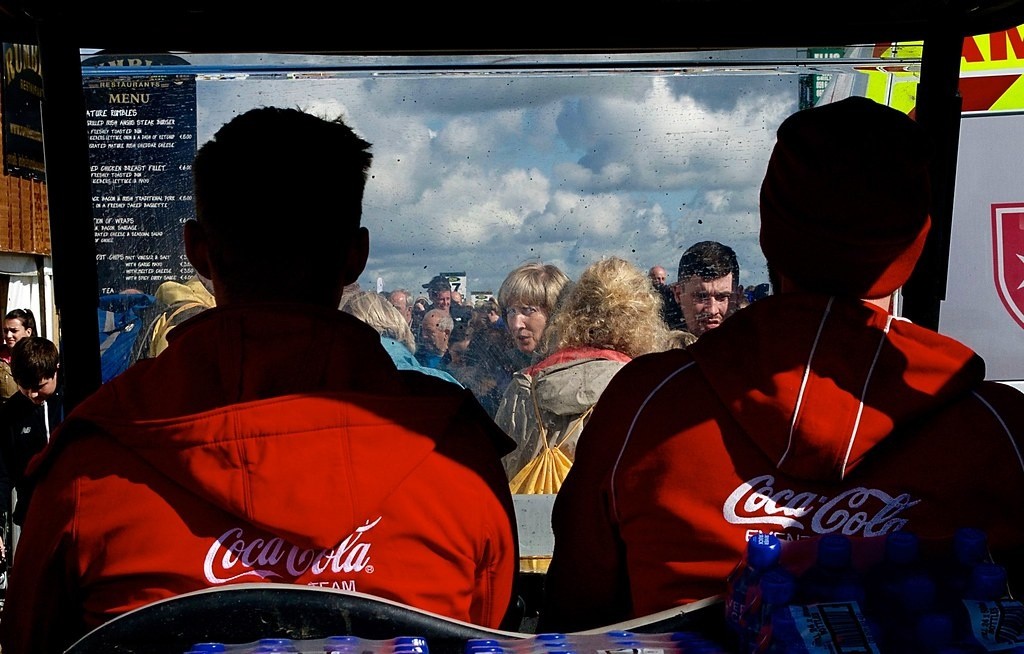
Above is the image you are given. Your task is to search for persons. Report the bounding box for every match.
[337,241,751,575]
[0,272,60,617]
[0,107,522,654]
[534,96,1024,635]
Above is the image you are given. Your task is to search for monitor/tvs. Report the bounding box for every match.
[36,0,961,627]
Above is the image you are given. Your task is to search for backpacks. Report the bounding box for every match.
[507,377,597,574]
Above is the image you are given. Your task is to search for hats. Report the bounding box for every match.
[759,97,932,300]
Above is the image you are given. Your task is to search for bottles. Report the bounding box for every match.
[725,527,1024,654]
[182,635,430,654]
[465,630,722,654]
